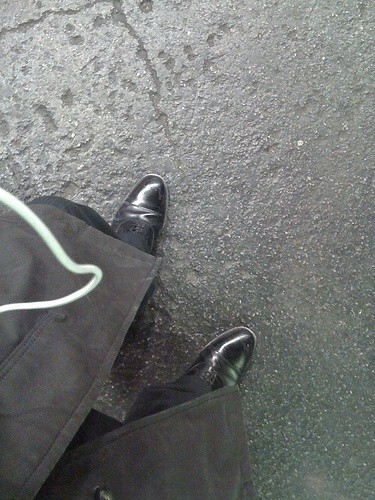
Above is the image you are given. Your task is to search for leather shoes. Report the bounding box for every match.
[112,172,168,262]
[183,324,256,390]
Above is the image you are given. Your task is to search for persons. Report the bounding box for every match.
[0,169,256,500]
